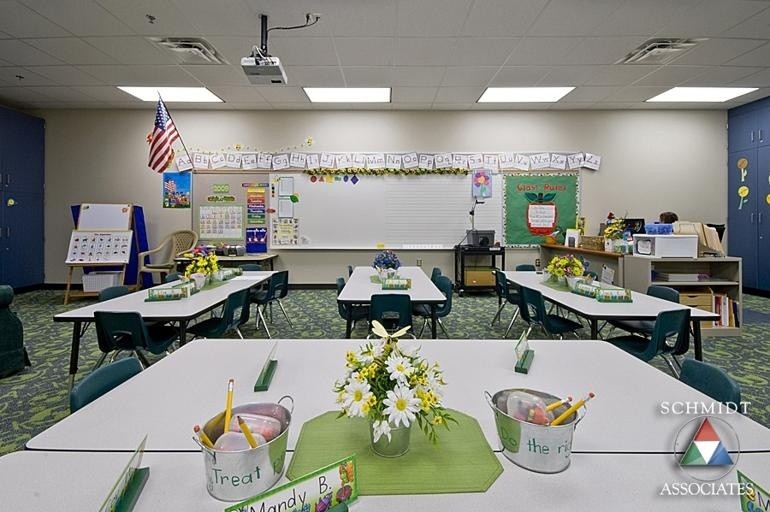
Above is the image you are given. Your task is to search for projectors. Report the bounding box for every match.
[239,55,288,87]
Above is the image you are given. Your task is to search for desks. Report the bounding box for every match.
[337,266,447,338]
[174,252,278,270]
[490,270,721,364]
[53,272,281,404]
[23,340,770,451]
[540,243,631,286]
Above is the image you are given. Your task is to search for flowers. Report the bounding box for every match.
[546,255,584,276]
[604,219,625,239]
[331,336,460,448]
[305,168,468,175]
[371,250,402,270]
[182,253,218,277]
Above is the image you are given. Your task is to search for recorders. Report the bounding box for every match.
[222,244,246,256]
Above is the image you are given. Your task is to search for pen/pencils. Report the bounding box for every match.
[236,415,258,450]
[223,378,234,434]
[193,425,214,450]
[551,392,595,426]
[545,395,573,411]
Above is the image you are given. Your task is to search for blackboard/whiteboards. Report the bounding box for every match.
[67,200,134,268]
[268,169,505,250]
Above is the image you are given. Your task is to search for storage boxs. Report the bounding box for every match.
[81,272,119,293]
[633,234,698,258]
[645,224,672,234]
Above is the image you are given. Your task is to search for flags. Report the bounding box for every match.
[147,97,179,174]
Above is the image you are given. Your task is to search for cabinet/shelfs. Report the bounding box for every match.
[728,95,769,291]
[624,256,743,336]
[455,244,505,296]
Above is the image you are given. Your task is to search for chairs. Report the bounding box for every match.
[427,267,441,283]
[606,285,679,335]
[415,277,451,338]
[338,279,369,321]
[71,356,145,413]
[186,290,250,340]
[371,294,415,335]
[490,270,520,338]
[136,231,198,290]
[679,358,740,413]
[349,266,353,276]
[605,309,690,379]
[249,271,295,338]
[585,270,599,280]
[96,286,131,302]
[165,272,184,283]
[90,310,179,372]
[520,287,583,340]
[517,265,536,271]
[238,264,263,272]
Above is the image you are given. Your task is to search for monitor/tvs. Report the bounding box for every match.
[621,218,646,234]
[706,223,726,242]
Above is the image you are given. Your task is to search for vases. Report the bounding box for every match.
[557,275,568,285]
[370,414,410,456]
